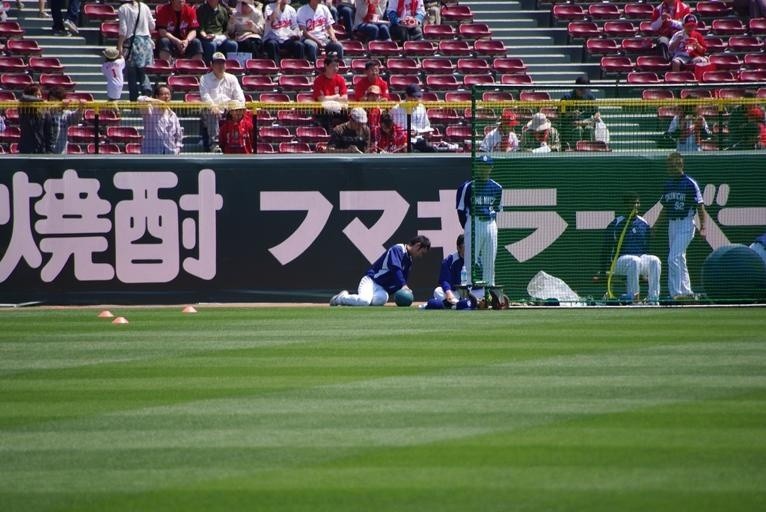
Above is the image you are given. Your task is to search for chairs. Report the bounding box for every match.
[0,0,766,154]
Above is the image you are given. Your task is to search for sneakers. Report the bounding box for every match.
[329,290,349,306]
[39,12,78,37]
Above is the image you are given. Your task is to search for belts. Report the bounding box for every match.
[479,217,491,220]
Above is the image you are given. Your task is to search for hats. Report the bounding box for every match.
[212,52,226,62]
[350,108,368,123]
[103,47,120,60]
[527,113,551,132]
[745,108,763,118]
[406,82,422,96]
[501,111,520,127]
[367,85,382,95]
[228,100,246,111]
[455,298,479,310]
[425,298,444,309]
[476,155,493,164]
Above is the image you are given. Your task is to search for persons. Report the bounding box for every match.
[136,84,183,154]
[155,0,442,60]
[101,48,125,101]
[15,84,86,154]
[601,191,663,306]
[15,0,80,36]
[329,236,428,308]
[556,75,598,145]
[651,0,708,70]
[421,234,479,310]
[658,153,708,301]
[668,90,765,150]
[482,110,561,151]
[117,0,155,104]
[457,155,502,309]
[198,51,253,153]
[313,57,436,152]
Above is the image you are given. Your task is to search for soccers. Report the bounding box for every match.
[394,289,413,306]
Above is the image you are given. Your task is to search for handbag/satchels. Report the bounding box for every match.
[415,138,456,152]
[427,5,441,25]
[121,46,132,62]
